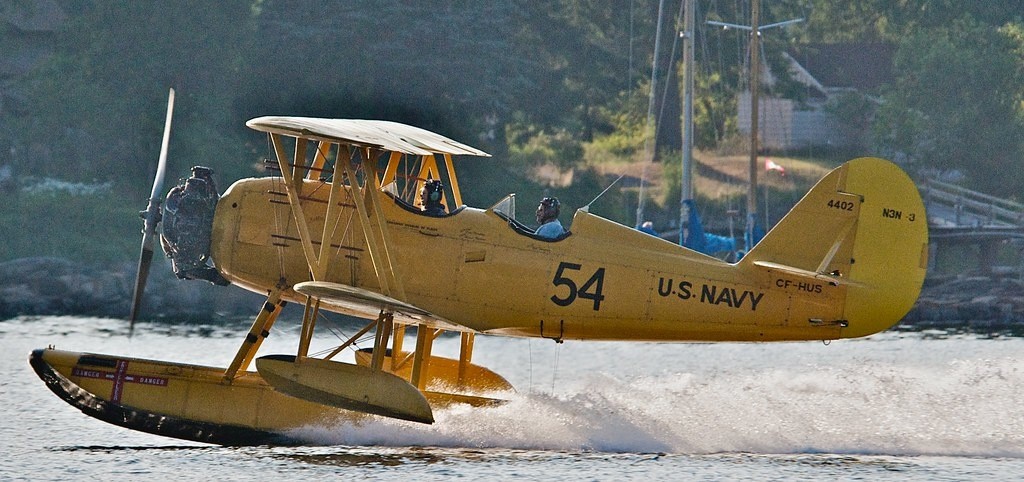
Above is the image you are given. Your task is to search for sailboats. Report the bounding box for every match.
[625,0,805,267]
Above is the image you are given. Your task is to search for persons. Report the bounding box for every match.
[420,180,447,214]
[534,197,564,238]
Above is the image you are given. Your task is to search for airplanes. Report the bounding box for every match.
[28,0,928,448]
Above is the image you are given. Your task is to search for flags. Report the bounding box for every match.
[766,158,784,177]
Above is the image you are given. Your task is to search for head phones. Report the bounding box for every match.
[547,198,556,217]
[431,180,440,201]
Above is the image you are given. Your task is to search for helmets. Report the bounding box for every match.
[540,196,560,219]
[423,180,443,202]
